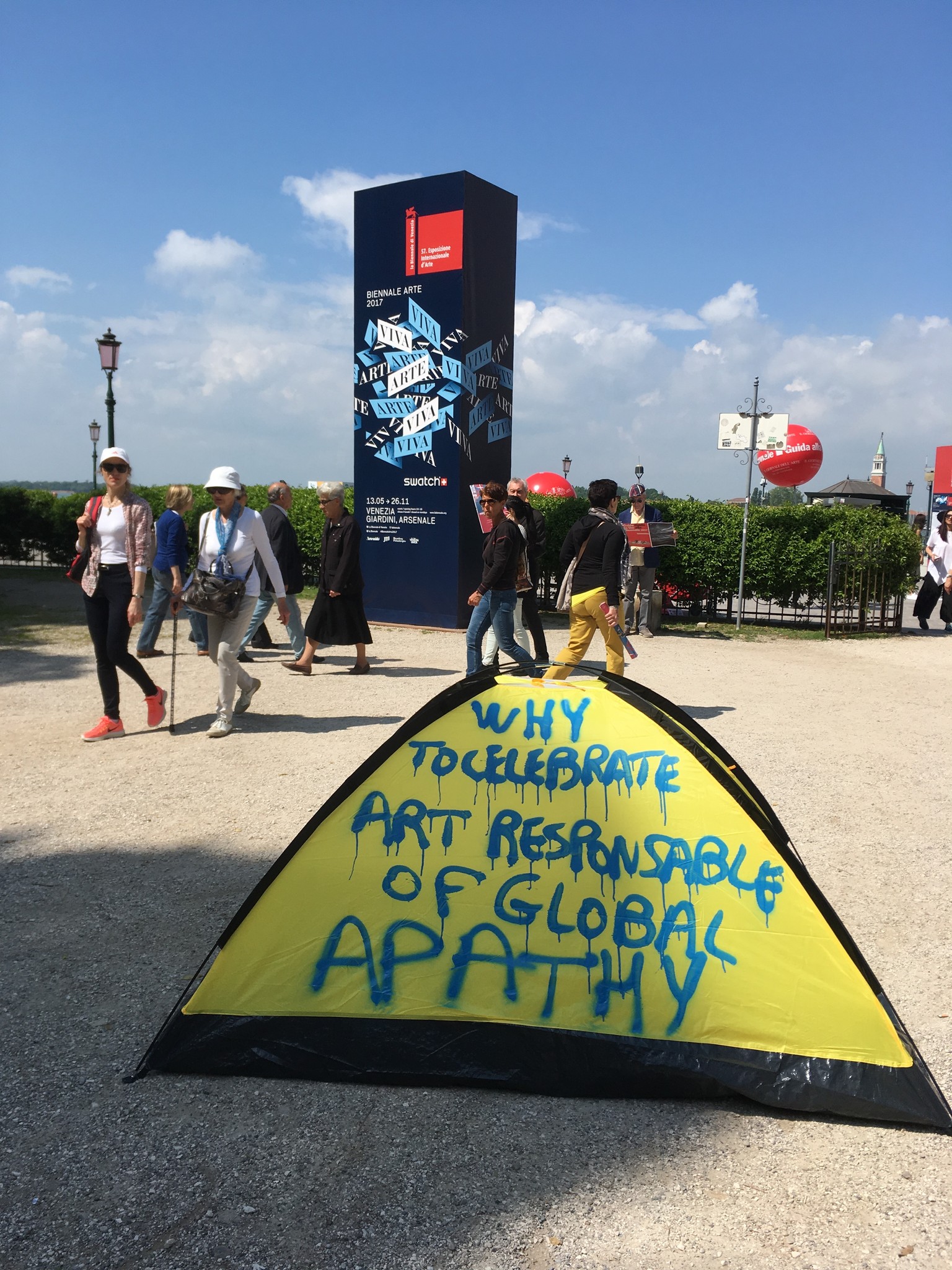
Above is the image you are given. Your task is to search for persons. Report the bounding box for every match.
[540,479,625,679]
[281,482,373,675]
[619,483,678,637]
[466,483,544,678]
[506,478,549,663]
[136,485,208,659]
[171,466,289,737]
[482,495,538,676]
[906,508,952,633]
[188,483,278,649]
[238,482,325,662]
[76,447,168,742]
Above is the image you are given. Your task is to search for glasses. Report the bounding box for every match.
[206,487,232,494]
[946,514,952,518]
[101,462,126,473]
[612,495,621,502]
[479,499,496,505]
[631,498,644,503]
[319,500,333,505]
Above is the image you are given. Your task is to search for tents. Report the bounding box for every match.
[124,661,952,1135]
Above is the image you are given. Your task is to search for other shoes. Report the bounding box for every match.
[296,655,324,663]
[349,663,370,675]
[281,661,312,675]
[510,658,548,675]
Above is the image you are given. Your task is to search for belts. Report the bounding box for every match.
[99,564,127,570]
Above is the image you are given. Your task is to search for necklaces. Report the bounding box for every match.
[106,489,127,515]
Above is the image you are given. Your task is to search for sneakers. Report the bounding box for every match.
[234,678,261,715]
[917,616,929,630]
[136,633,281,662]
[81,716,125,742]
[205,719,233,736]
[143,686,168,728]
[944,624,952,633]
[623,629,653,637]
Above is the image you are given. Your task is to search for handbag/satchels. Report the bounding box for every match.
[66,496,102,586]
[179,558,246,619]
[555,557,577,614]
[516,578,532,593]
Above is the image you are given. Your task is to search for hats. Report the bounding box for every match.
[98,447,130,467]
[203,466,241,490]
[628,483,645,497]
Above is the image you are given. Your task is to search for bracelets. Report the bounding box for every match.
[609,605,618,610]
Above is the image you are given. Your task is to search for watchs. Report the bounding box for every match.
[476,590,482,597]
[132,594,144,599]
[946,574,952,577]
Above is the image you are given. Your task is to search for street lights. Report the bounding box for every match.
[562,455,572,479]
[905,480,915,522]
[94,326,122,448]
[88,418,102,490]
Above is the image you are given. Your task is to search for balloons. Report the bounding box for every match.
[757,424,823,487]
[525,472,577,498]
